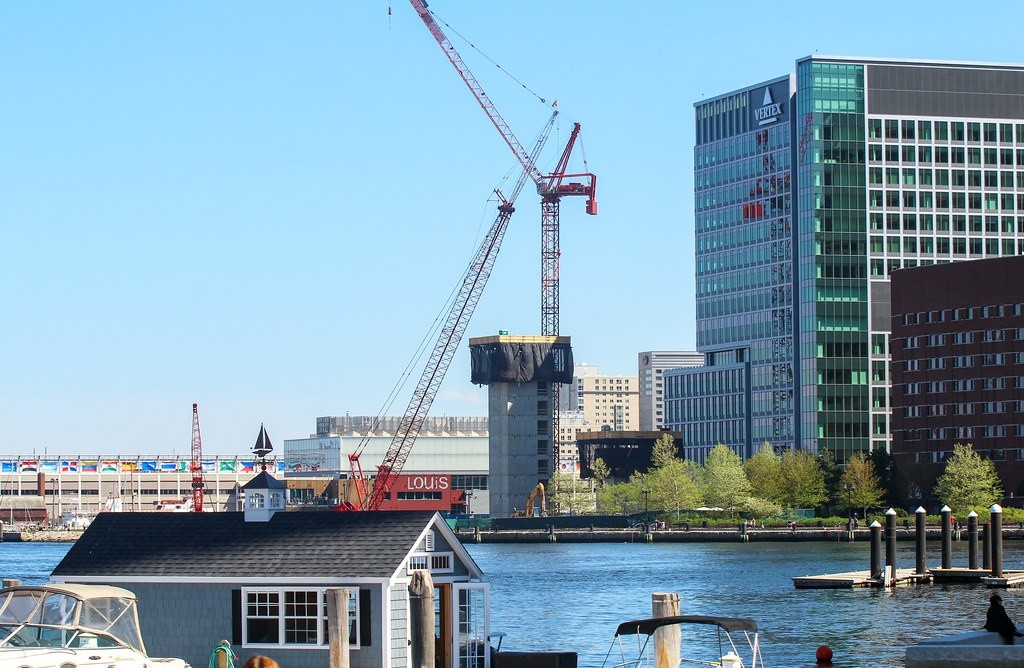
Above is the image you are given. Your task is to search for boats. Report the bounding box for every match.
[602,616,768,668]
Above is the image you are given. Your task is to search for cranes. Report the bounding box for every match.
[388,1,599,525]
[745,110,816,457]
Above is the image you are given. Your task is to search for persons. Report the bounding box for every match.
[654,517,871,531]
[1010,491,1014,497]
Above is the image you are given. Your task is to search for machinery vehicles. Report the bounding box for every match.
[509,482,548,518]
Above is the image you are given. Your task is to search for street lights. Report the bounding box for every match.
[844,482,855,532]
[50,478,58,530]
[464,491,474,528]
[641,488,652,531]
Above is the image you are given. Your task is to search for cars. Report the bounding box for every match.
[0,584,190,668]
